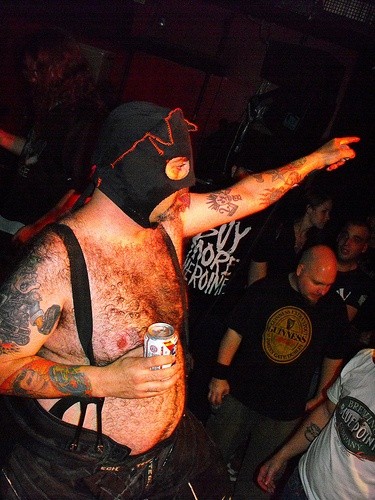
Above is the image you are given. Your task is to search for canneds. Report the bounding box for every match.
[144,323,178,370]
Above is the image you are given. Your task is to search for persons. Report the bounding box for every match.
[181,148,375,500]
[0,100,361,500]
[0,31,108,262]
